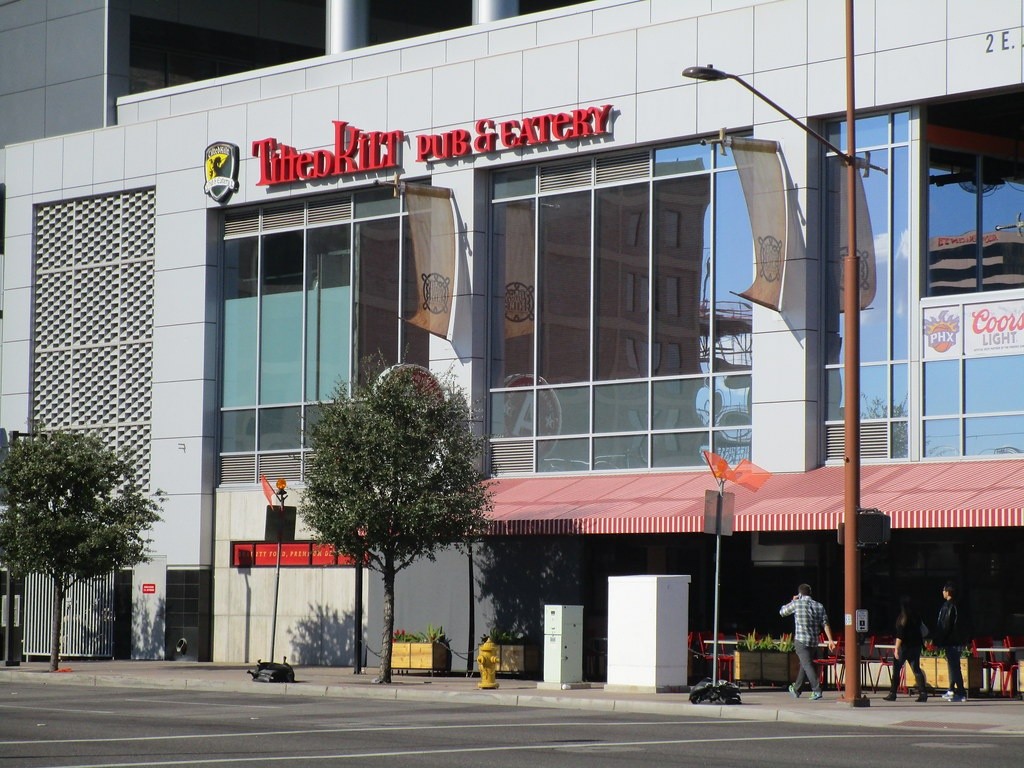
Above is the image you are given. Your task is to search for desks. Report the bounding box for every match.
[873,644,896,648]
[817,640,838,689]
[588,637,607,681]
[976,646,1024,698]
[703,639,745,650]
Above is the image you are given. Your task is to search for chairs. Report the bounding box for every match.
[688,631,908,694]
[972,635,1019,698]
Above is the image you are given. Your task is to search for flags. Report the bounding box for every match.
[704,450,737,484]
[262,476,274,511]
[729,460,770,493]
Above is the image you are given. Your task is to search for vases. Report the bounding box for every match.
[478,644,539,680]
[391,642,452,677]
[905,656,983,698]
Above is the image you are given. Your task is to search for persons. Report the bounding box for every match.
[883,595,927,702]
[932,583,967,703]
[780,584,836,700]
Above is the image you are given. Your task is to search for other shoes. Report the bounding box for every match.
[915,694,927,702]
[954,695,967,702]
[809,691,822,700]
[883,693,896,701]
[789,685,799,699]
[942,691,954,699]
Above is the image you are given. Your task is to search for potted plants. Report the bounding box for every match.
[733,630,796,692]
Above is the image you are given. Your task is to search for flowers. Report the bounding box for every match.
[919,640,972,658]
[479,627,530,645]
[392,624,445,643]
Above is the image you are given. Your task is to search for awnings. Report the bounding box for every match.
[463,458,1024,535]
